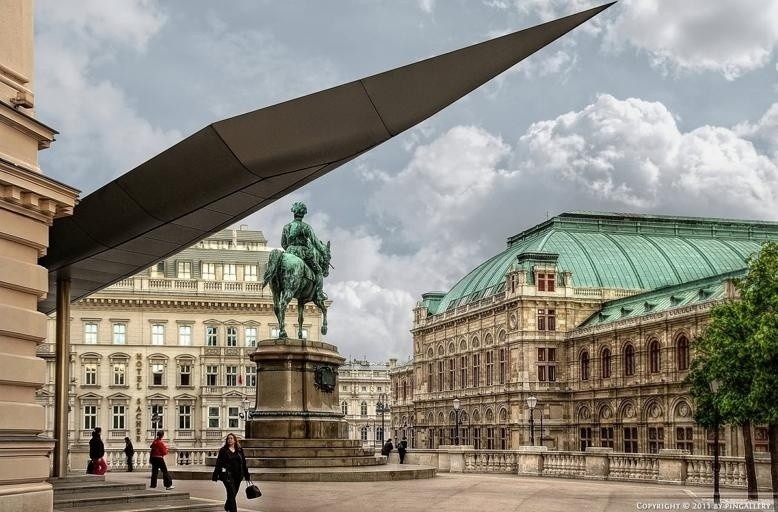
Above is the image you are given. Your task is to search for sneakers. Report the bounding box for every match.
[166,486,176,490]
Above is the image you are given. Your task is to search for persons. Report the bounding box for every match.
[211,432,251,512]
[89,430,96,460]
[280,201,331,301]
[398,436,408,464]
[123,436,135,471]
[381,439,394,456]
[149,430,176,490]
[92,426,108,475]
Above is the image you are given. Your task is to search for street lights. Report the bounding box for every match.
[709,376,723,505]
[376,393,390,449]
[526,394,537,444]
[452,397,461,444]
[151,413,160,438]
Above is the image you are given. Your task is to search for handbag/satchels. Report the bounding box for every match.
[246,480,262,499]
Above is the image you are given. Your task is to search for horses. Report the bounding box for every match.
[261,240,332,340]
